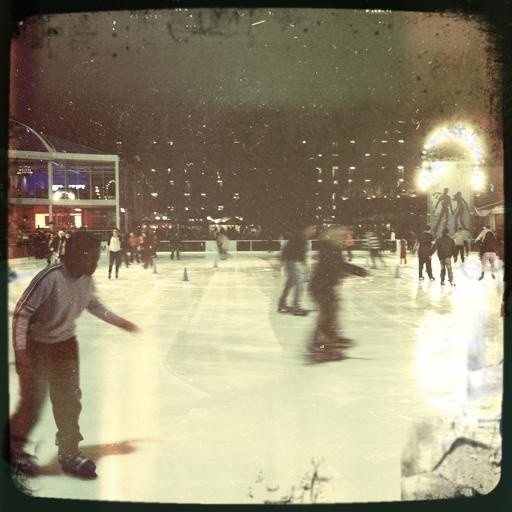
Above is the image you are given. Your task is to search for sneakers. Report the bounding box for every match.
[10,450,39,478]
[278,300,306,312]
[310,339,349,361]
[61,451,96,478]
[454,251,468,262]
[419,276,455,287]
[478,272,495,280]
[109,272,118,278]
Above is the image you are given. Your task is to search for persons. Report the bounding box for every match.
[276,220,317,317]
[451,191,469,232]
[12,219,276,280]
[1,232,143,476]
[298,226,373,366]
[340,221,505,292]
[431,187,454,233]
